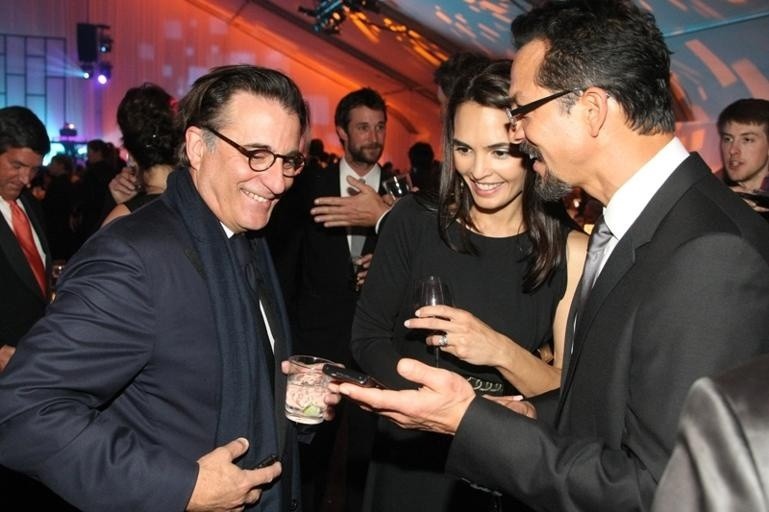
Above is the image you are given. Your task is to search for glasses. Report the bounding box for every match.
[193,122,307,178]
[504,83,596,126]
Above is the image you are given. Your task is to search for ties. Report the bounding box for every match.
[350,177,368,274]
[7,197,48,303]
[575,212,615,337]
[231,234,267,308]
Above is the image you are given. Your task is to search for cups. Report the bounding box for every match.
[382,173,413,203]
[283,355,337,427]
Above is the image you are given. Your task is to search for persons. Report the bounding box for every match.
[2,1,769,512]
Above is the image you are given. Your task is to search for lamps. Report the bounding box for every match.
[77,22,114,85]
[299,0,378,37]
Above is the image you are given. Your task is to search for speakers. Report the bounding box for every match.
[76,22,103,62]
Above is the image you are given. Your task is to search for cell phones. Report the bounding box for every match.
[321,364,388,391]
[256,453,278,472]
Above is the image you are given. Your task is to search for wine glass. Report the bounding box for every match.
[418,276,456,371]
